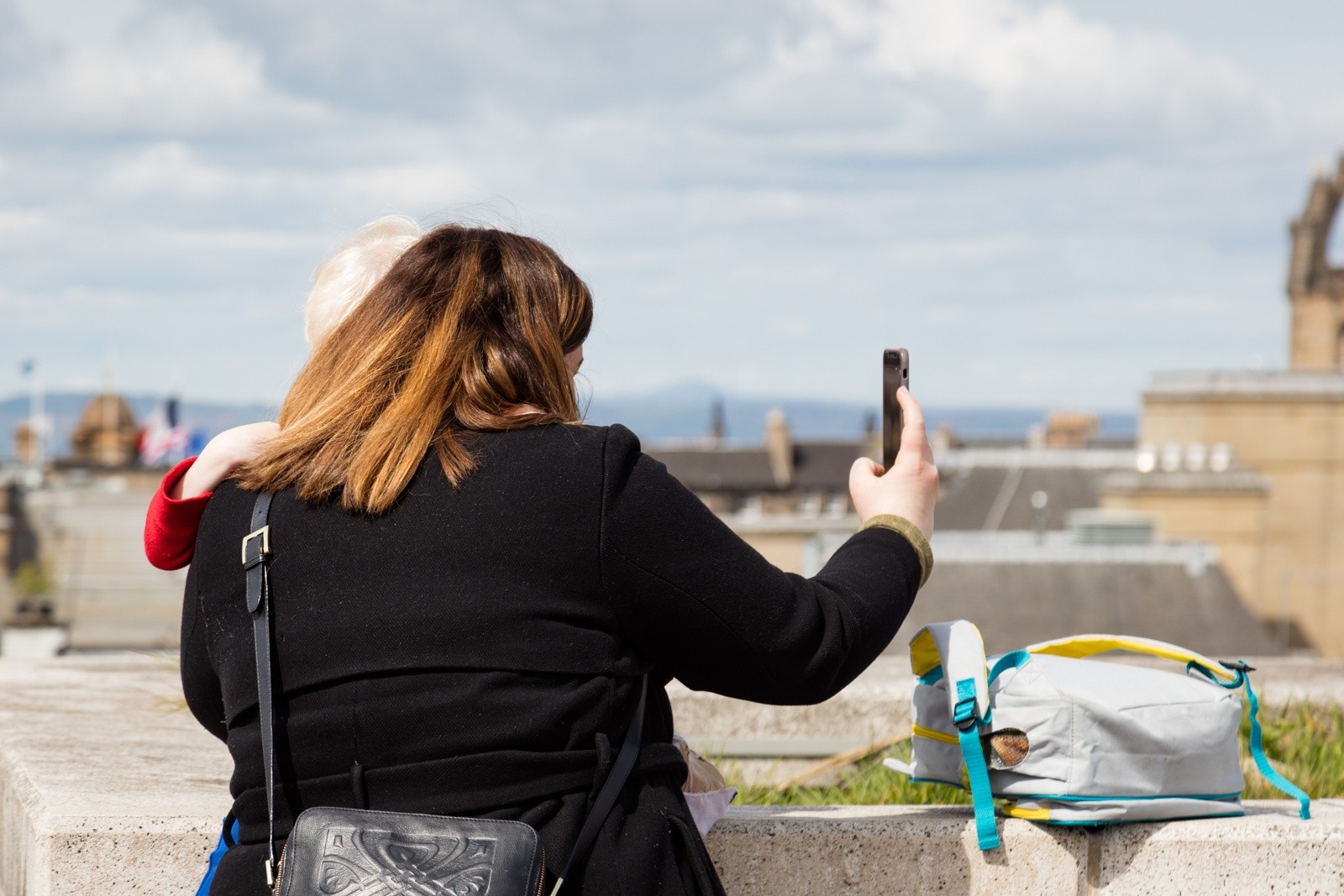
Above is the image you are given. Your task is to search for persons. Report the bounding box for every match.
[144,214,424,572]
[178,224,939,896]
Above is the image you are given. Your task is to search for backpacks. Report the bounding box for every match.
[909,619,1311,851]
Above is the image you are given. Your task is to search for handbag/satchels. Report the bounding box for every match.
[242,492,648,896]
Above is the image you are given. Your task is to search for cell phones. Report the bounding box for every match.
[882,349,909,470]
